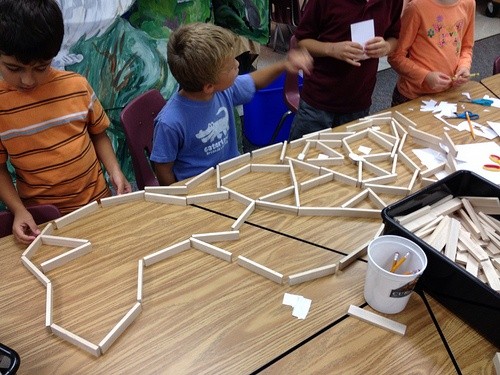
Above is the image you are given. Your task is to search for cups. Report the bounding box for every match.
[363,235,428,314]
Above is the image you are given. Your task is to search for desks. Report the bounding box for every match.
[0,77,500,375]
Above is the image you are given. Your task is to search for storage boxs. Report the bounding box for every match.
[243,69,303,145]
[379,169,500,348]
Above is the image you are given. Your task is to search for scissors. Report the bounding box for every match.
[444,110,479,119]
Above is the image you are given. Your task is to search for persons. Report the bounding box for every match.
[288,0,404,142]
[388,0,476,107]
[150,22,313,186]
[0,0,132,247]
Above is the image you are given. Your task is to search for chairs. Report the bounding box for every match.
[120,90,167,189]
[270,35,307,145]
[0,205,60,239]
[268,0,303,51]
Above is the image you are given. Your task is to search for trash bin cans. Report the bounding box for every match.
[244,72,303,144]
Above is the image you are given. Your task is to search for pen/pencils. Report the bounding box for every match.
[392,253,399,268]
[465,110,475,139]
[403,269,420,275]
[391,251,412,272]
